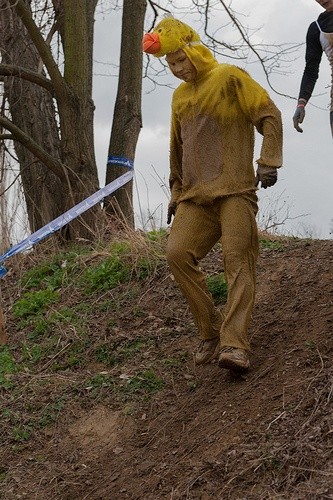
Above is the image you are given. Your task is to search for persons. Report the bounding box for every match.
[142,17,282,372]
[293,0,333,138]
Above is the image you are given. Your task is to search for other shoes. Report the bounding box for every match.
[195,335,219,365]
[219,346,250,374]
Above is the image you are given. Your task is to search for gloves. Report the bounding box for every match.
[254,163,277,189]
[293,100,307,133]
[167,192,182,224]
